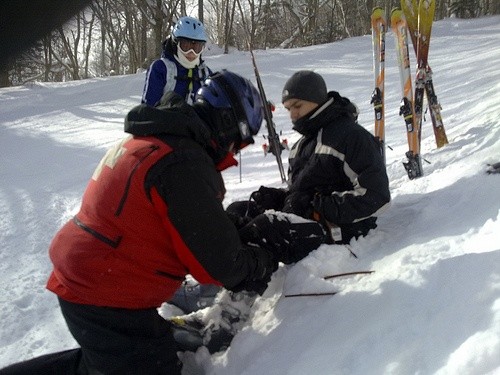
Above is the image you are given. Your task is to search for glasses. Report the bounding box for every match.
[236,117,254,153]
[178,38,206,56]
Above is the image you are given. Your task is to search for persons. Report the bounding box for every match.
[0,16,280,375]
[166,70,391,356]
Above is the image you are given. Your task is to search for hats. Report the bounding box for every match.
[282,71,327,104]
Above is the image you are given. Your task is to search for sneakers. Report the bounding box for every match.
[166,291,255,350]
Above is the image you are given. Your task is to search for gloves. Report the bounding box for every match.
[244,246,280,297]
[251,186,287,212]
[282,192,314,220]
[159,91,184,107]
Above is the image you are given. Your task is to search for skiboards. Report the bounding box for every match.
[369,6,421,180]
[401,1,449,149]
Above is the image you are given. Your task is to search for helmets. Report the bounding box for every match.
[196,70,264,135]
[172,17,207,44]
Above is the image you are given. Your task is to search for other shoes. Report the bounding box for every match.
[165,281,213,311]
